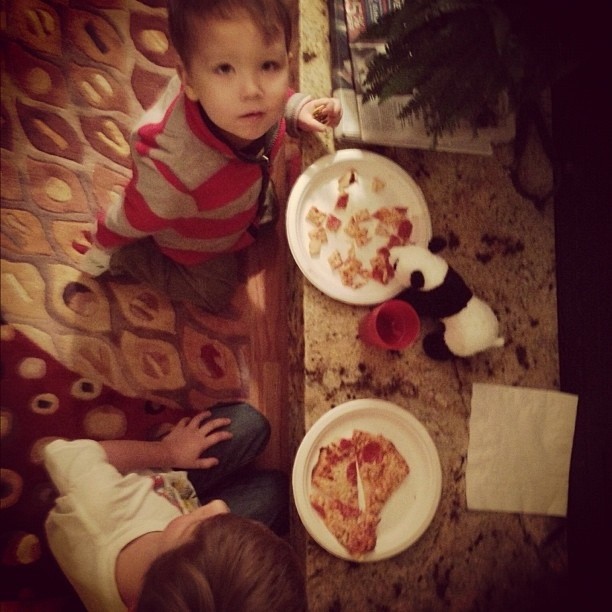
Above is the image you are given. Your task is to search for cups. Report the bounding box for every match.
[358,302,422,353]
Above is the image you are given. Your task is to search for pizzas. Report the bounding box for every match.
[309,429,410,555]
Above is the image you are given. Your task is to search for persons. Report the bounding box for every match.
[70,1,343,283]
[41,404,306,612]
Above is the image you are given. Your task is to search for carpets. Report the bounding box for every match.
[3,0,289,611]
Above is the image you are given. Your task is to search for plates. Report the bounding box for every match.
[291,398,442,563]
[285,148,432,308]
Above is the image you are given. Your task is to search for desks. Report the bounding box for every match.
[284,0,570,612]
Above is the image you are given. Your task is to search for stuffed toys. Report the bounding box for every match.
[385,238,506,361]
